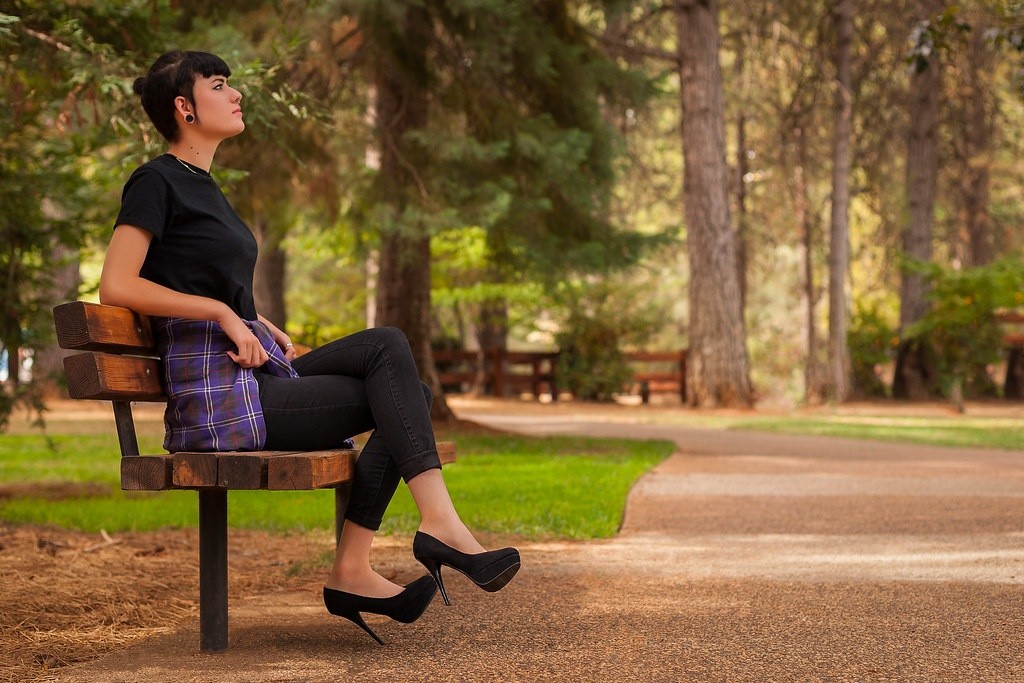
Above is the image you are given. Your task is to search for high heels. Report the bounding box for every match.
[413,531,521,606]
[323,575,439,645]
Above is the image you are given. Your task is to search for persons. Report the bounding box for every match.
[100,51,521,646]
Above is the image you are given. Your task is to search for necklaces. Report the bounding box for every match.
[173,155,213,179]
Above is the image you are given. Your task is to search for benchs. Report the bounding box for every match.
[431,349,686,403]
[52,301,457,653]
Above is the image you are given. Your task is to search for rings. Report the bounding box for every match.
[282,343,292,349]
